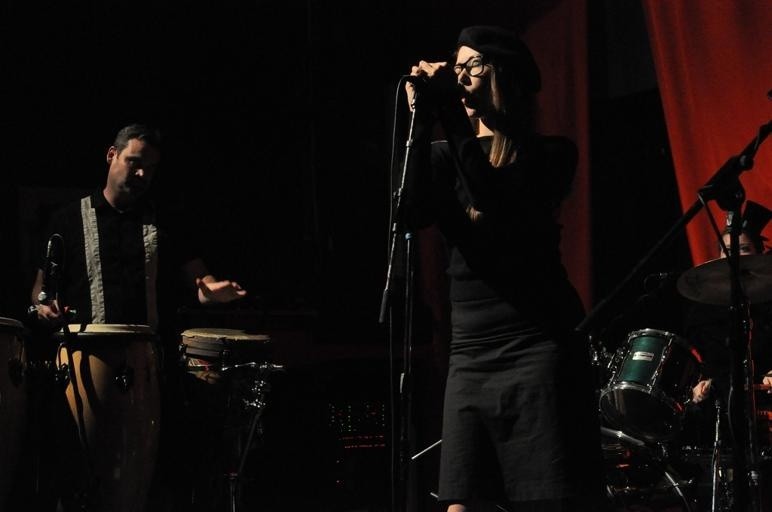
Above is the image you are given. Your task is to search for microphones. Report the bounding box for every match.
[41,232,66,294]
[407,74,469,99]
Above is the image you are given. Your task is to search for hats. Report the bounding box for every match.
[458,25,542,94]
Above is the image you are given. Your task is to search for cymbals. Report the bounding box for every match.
[677,255,772,307]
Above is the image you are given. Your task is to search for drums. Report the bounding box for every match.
[55,322,164,512]
[1,316,29,510]
[598,327,772,512]
[183,326,274,385]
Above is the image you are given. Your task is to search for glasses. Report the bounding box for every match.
[453,56,484,77]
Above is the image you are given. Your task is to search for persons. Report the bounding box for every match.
[29,117,251,511]
[386,11,601,512]
[683,220,772,434]
